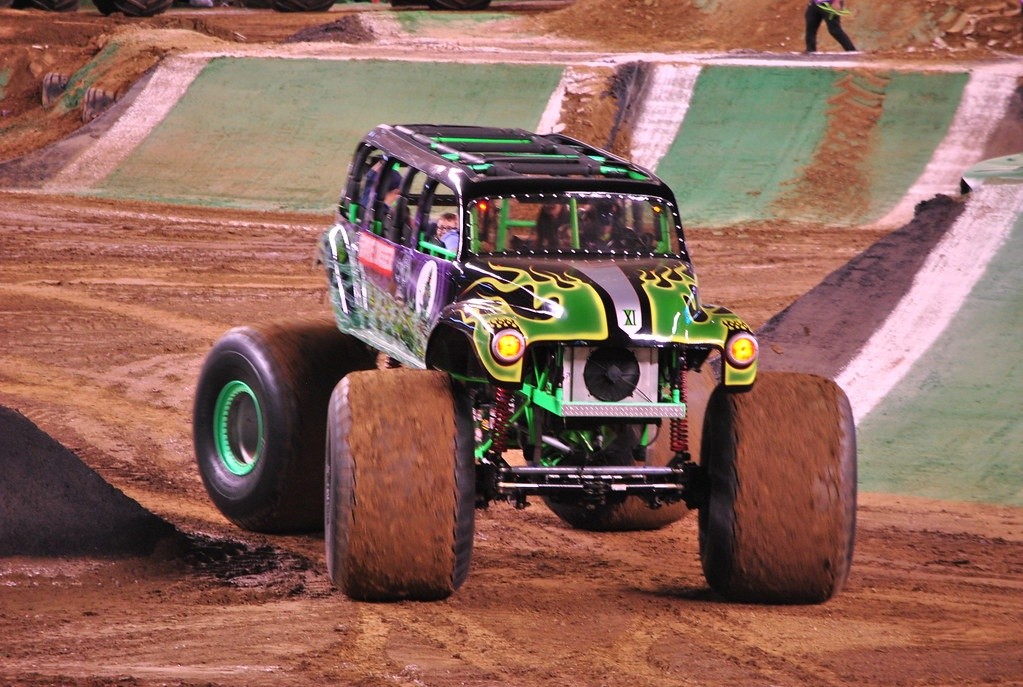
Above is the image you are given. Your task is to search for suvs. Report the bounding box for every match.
[194,123,857,607]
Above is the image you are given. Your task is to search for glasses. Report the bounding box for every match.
[578,208,596,218]
[437,225,457,232]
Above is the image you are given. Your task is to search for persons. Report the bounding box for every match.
[354,156,412,234]
[529,200,657,254]
[480,206,523,254]
[805,0,859,53]
[428,212,458,258]
